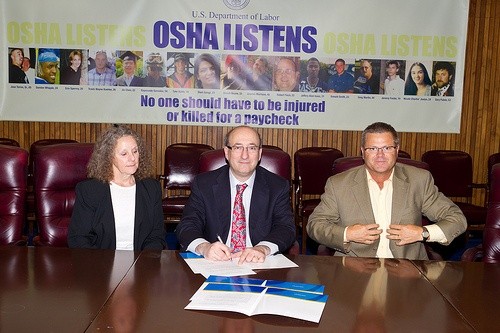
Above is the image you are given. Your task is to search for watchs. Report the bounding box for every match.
[421,227,430,242]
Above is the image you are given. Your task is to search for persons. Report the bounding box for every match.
[176,126,297,264]
[68,124,164,249]
[9,46,455,97]
[306,122,468,260]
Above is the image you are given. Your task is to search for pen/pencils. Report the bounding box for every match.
[214,232,233,263]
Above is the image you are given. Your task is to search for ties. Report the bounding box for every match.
[229,183,248,253]
[438,90,442,96]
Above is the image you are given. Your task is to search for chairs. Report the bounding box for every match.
[0,137,500,264]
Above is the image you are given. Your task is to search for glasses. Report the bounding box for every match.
[275,68,297,75]
[227,144,261,152]
[363,145,397,154]
[149,66,163,71]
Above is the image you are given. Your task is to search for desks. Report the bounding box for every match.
[0,244,500,333]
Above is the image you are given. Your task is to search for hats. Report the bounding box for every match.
[145,53,163,64]
[119,51,141,64]
[38,51,58,63]
[175,54,186,61]
